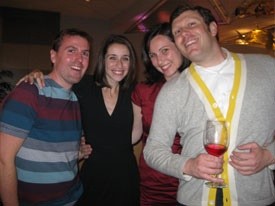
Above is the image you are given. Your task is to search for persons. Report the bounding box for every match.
[142,5,275,206]
[16,21,192,206]
[0,28,93,206]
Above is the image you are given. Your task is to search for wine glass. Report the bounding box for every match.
[203,120,230,188]
